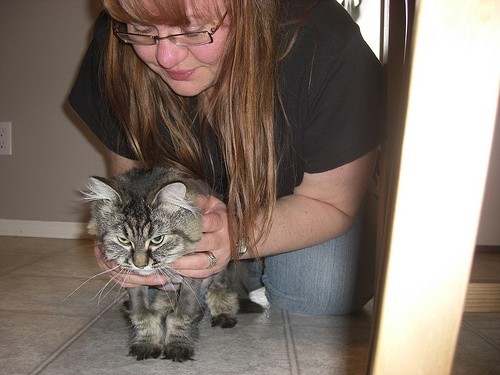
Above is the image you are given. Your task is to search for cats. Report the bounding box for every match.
[64,166,238,363]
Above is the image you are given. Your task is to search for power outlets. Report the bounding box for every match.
[1,122,13,155]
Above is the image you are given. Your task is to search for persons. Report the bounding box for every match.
[66,0,387,316]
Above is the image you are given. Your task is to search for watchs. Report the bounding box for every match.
[235,237,248,256]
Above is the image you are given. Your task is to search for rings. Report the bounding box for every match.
[207,252,216,268]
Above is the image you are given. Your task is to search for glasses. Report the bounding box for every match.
[113,9,230,47]
